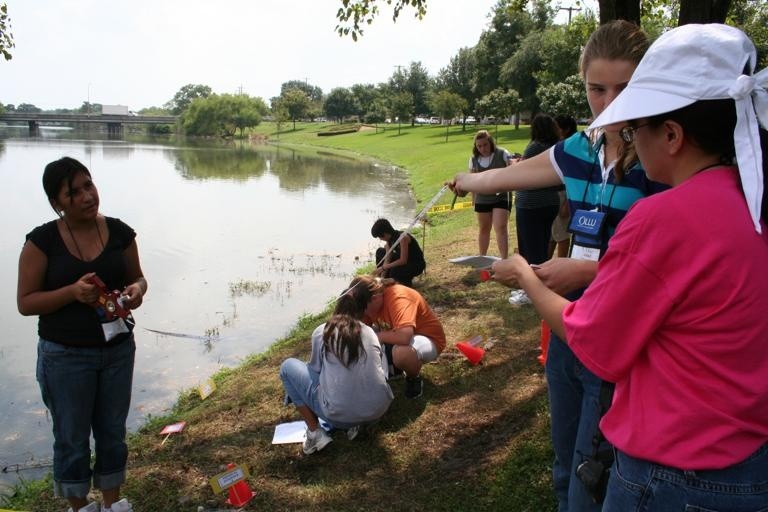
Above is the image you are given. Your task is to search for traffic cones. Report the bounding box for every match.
[454,340,485,365]
[535,318,549,366]
[477,266,492,283]
[222,462,258,509]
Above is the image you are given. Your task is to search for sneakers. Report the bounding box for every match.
[406,376,424,399]
[346,425,364,442]
[302,427,334,455]
[508,287,533,308]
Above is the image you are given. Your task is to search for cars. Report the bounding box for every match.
[577,117,589,125]
[382,114,504,126]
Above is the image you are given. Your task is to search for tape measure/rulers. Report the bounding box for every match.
[85,182,450,341]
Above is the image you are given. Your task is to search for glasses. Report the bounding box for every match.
[618,122,652,144]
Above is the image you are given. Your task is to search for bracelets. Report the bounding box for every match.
[381,265,386,271]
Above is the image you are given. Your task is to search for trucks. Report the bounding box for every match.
[100,103,138,116]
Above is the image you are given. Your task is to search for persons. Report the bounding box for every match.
[279,289,396,455]
[16,157,148,512]
[370,218,426,287]
[349,276,446,403]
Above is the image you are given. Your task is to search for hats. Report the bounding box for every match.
[589,23,764,132]
